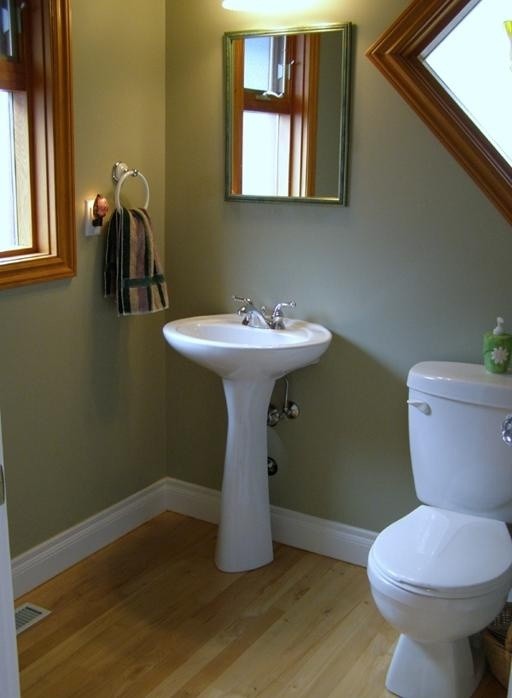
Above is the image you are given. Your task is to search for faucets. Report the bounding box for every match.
[236,306,253,322]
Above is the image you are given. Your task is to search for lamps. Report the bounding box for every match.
[91,191,109,227]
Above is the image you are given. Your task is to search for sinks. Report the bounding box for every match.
[163,312,333,380]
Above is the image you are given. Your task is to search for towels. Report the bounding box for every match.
[102,209,171,320]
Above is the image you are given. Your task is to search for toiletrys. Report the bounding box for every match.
[483,316,512,373]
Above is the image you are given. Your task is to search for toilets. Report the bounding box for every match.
[366,359,512,698]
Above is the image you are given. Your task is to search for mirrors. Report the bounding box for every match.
[223,22,353,207]
